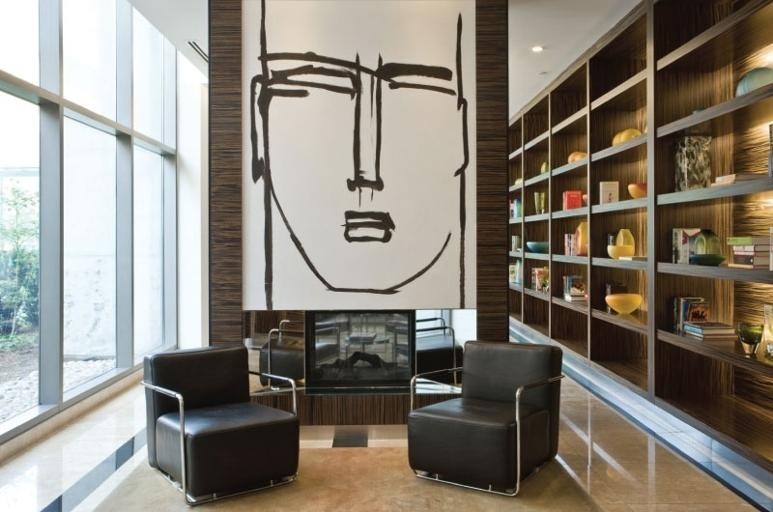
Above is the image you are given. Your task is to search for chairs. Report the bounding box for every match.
[402,337,561,498]
[141,346,300,504]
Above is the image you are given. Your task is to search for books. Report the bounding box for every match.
[600,181,619,204]
[725,227,773,272]
[672,296,738,340]
[564,234,575,256]
[563,191,582,210]
[562,275,586,302]
[711,174,768,186]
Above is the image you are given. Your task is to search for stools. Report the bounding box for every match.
[259,321,339,384]
[416,335,464,383]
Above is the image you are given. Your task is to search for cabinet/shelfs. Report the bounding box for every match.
[503,0,772,512]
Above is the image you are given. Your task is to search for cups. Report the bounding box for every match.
[534,191,548,215]
